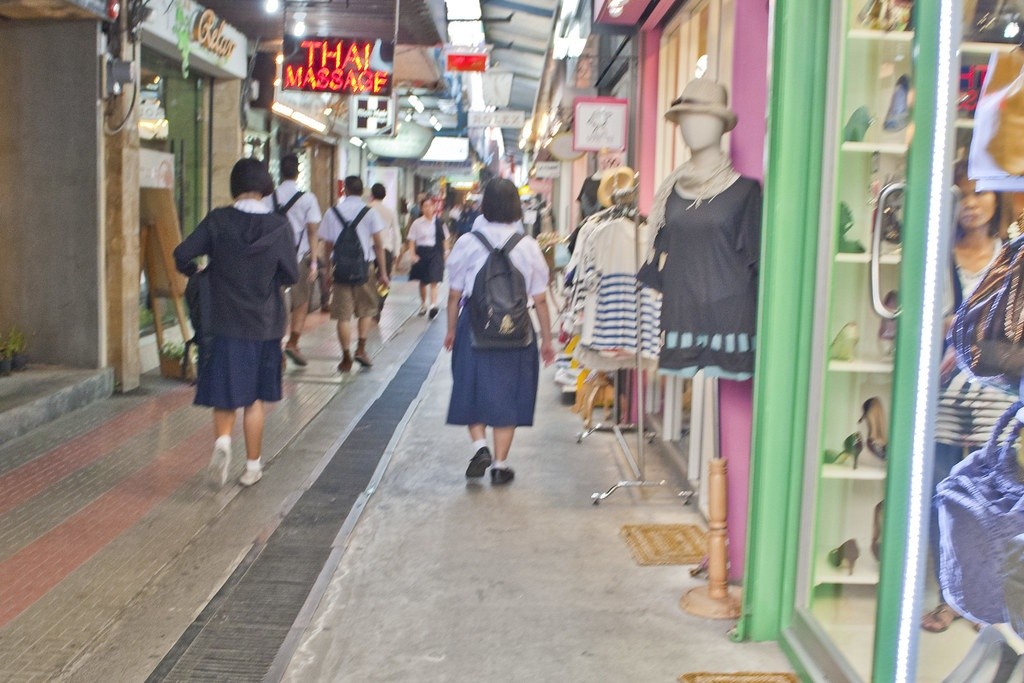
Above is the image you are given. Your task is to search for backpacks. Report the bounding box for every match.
[467,231,532,349]
[331,206,368,285]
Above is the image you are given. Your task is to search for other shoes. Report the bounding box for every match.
[839,203,864,254]
[285,344,307,366]
[338,356,354,371]
[465,447,491,479]
[871,205,902,244]
[428,306,438,320]
[239,470,263,486]
[206,437,232,490]
[490,466,514,485]
[418,307,427,317]
[355,349,370,366]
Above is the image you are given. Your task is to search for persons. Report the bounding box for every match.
[267,155,401,372]
[443,176,555,482]
[636,79,762,379]
[406,189,552,319]
[919,158,1018,633]
[169,156,299,490]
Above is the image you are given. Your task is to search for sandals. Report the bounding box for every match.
[922,601,961,633]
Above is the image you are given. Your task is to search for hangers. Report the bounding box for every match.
[596,199,646,228]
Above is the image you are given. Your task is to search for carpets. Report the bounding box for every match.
[623,522,710,567]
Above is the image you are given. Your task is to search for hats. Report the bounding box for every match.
[665,78,739,134]
[597,163,636,208]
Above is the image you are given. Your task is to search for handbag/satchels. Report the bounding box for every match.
[931,41,1024,641]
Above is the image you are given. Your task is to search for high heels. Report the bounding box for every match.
[828,539,859,575]
[884,73,911,132]
[841,106,870,143]
[879,290,898,340]
[828,323,860,363]
[871,498,885,559]
[858,397,889,458]
[824,433,861,469]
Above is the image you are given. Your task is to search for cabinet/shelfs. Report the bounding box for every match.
[809,27,913,597]
[952,41,1019,126]
